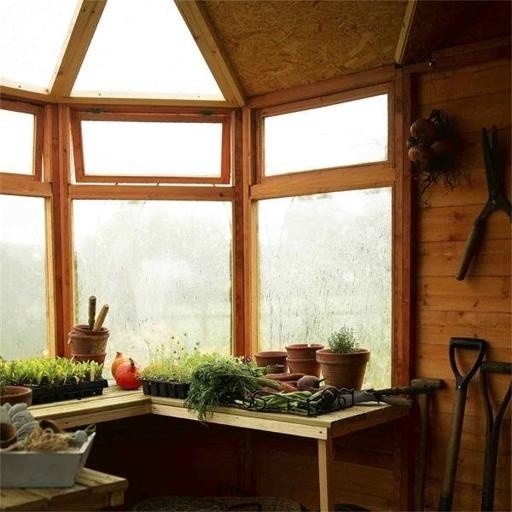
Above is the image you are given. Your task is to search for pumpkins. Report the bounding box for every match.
[112,352,132,375]
[114,358,142,389]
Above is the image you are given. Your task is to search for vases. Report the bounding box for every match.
[253,343,324,379]
[0,385,34,410]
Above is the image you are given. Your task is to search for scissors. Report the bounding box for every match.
[456,124,512,281]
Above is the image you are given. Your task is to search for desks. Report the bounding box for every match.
[0,374,419,512]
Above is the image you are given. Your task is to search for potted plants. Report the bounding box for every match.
[316,323,370,392]
[0,331,245,406]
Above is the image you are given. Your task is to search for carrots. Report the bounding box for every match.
[246,373,305,398]
